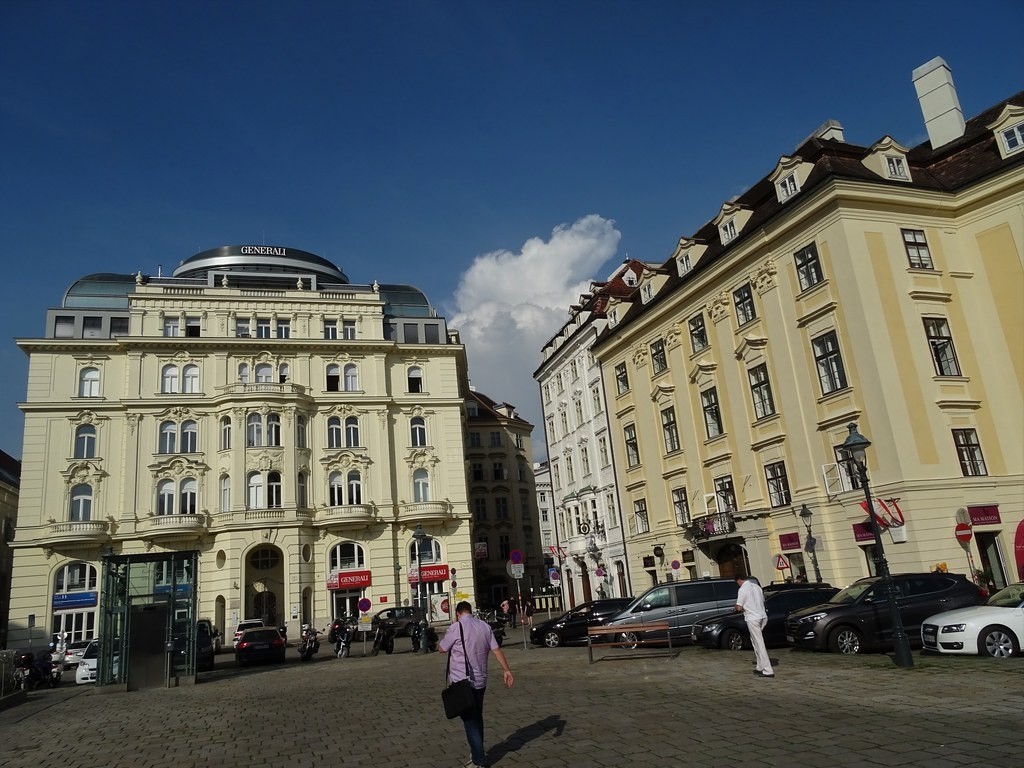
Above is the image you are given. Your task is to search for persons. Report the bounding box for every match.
[770,571,809,585]
[734,573,775,677]
[436,601,512,768]
[499,597,535,629]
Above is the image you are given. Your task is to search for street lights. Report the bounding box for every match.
[411,522,427,619]
[841,422,914,668]
[799,503,823,583]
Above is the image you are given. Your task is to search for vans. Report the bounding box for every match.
[604,575,762,650]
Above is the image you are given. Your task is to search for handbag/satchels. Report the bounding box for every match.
[441,679,475,719]
[531,605,536,612]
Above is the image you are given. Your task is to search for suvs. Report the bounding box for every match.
[355,606,428,642]
[691,580,842,651]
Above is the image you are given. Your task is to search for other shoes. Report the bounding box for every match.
[510,625,512,628]
[753,670,762,674]
[463,760,486,768]
[469,751,489,759]
[759,674,774,677]
[513,625,517,629]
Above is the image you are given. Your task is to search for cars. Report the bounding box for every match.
[233,618,285,666]
[921,583,1024,658]
[171,618,224,672]
[327,616,358,643]
[64,637,119,684]
[530,597,649,648]
[786,570,990,655]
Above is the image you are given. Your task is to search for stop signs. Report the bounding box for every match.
[955,523,972,543]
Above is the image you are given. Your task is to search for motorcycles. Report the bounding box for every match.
[14,641,66,690]
[333,615,357,659]
[298,622,326,663]
[371,615,397,656]
[278,621,289,645]
[409,608,420,651]
[486,613,510,647]
